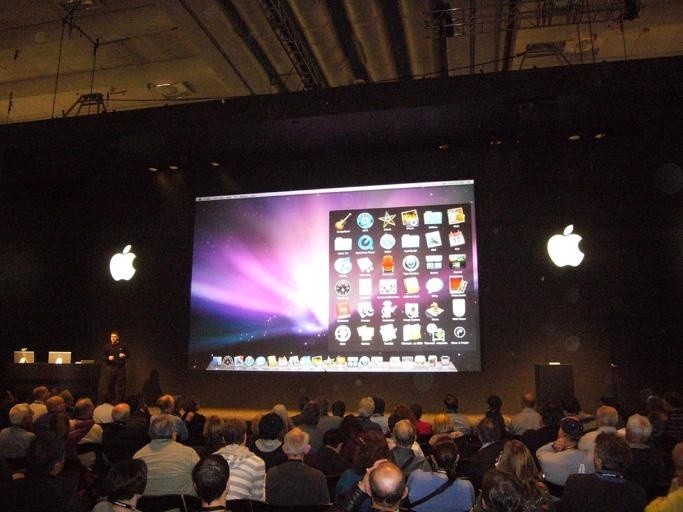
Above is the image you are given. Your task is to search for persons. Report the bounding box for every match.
[99,328,132,405]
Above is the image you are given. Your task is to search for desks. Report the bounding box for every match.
[0,360,100,412]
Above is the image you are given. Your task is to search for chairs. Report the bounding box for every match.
[452,423,674,510]
[69,425,347,510]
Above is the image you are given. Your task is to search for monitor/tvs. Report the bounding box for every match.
[13,351,35,364]
[47,351,72,364]
